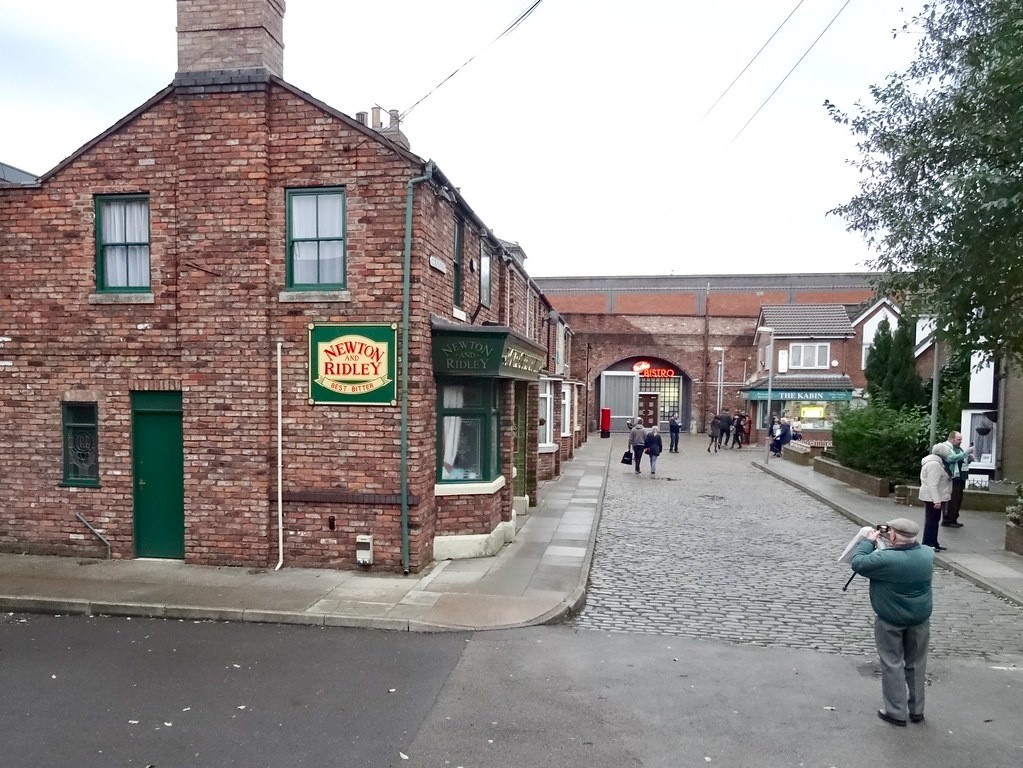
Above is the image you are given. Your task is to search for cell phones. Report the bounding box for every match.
[877,525,889,533]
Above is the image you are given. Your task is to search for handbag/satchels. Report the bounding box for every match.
[644,448,651,454]
[620,449,633,465]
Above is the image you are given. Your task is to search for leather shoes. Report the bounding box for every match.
[932,545,947,552]
[908,713,924,723]
[878,709,907,727]
[941,521,963,528]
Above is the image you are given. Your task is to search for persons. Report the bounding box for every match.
[769,416,805,440]
[850,518,935,728]
[644,426,662,475]
[918,443,955,552]
[668,412,683,453]
[627,417,641,429]
[734,409,752,445]
[769,417,791,457]
[940,430,976,528]
[706,415,722,453]
[729,412,743,449]
[718,407,734,449]
[628,418,647,474]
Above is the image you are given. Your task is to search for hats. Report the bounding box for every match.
[886,517,920,538]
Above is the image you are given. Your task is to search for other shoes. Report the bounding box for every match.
[714,449,717,452]
[707,448,711,452]
[718,444,721,449]
[730,446,734,449]
[737,446,742,449]
[636,471,641,474]
[670,451,679,453]
[651,472,655,475]
